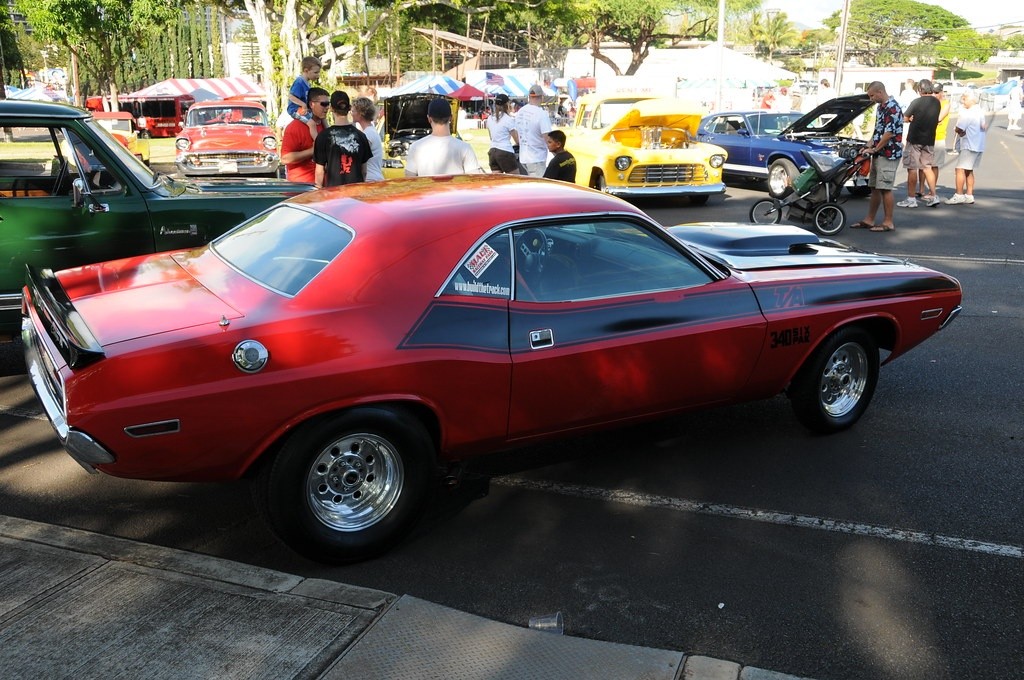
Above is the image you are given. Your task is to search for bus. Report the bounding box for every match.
[84,93,197,139]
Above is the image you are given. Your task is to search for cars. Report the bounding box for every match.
[547,91,730,207]
[20,174,964,570]
[371,93,492,179]
[692,94,876,199]
[60,111,152,169]
[0,98,325,332]
[175,100,280,180]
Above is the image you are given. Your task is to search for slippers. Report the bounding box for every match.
[849,220,875,229]
[870,224,894,232]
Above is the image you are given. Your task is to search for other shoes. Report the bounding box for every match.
[1012,125,1021,130]
[923,195,930,198]
[915,192,925,196]
[1007,125,1012,130]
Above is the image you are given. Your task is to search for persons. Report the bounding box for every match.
[896,79,987,208]
[850,81,904,233]
[761,79,838,126]
[515,85,556,177]
[1007,79,1024,130]
[276,57,386,189]
[543,130,577,183]
[404,98,485,177]
[479,101,576,127]
[487,94,519,174]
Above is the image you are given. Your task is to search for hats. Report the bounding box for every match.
[933,83,943,92]
[331,90,350,108]
[495,94,510,101]
[529,84,546,96]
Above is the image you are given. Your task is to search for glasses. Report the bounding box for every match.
[312,101,330,107]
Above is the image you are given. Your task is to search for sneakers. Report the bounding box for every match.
[926,195,940,207]
[945,193,966,204]
[897,197,918,207]
[962,193,974,204]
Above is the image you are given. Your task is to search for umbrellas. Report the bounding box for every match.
[4,85,63,102]
[377,74,560,112]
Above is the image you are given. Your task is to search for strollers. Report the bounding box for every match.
[749,146,871,236]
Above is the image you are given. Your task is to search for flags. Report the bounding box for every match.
[486,72,504,86]
